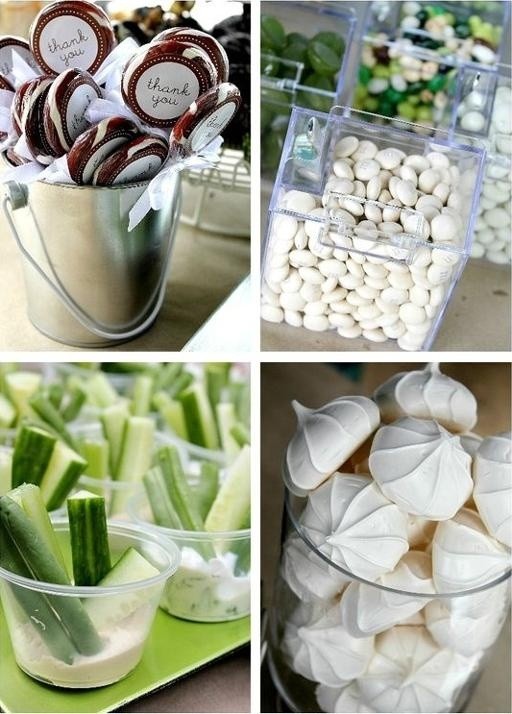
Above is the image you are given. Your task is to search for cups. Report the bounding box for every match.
[1,408,250,691]
[262,492,507,711]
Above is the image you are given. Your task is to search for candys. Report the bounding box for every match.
[285,363,512,713]
[261,0,512,351]
[1,1,244,187]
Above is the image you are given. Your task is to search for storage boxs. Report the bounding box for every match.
[261,100,489,352]
[261,1,365,195]
[428,57,511,272]
[352,1,510,128]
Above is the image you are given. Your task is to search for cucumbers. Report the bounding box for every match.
[1,363,250,663]
